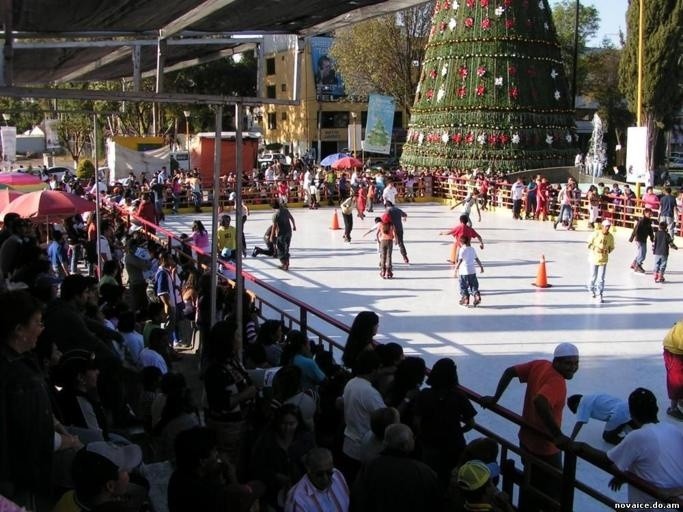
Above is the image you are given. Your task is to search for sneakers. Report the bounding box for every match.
[169,338,193,350]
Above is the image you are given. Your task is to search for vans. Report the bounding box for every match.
[170,151,188,173]
[10,167,42,182]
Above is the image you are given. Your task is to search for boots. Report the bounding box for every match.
[630,260,644,272]
[459,293,482,305]
[380,270,393,277]
[653,272,665,281]
[402,255,408,263]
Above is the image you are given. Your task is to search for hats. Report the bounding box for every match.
[602,220,612,227]
[73,441,143,487]
[35,273,63,287]
[228,192,236,200]
[12,219,28,227]
[457,459,500,491]
[60,349,98,370]
[554,343,579,358]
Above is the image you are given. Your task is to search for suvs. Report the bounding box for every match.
[258,153,288,166]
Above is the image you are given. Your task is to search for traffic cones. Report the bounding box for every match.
[529,252,556,287]
[326,208,342,230]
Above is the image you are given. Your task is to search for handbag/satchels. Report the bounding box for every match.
[146,282,161,305]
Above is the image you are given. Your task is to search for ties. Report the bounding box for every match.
[221,248,231,258]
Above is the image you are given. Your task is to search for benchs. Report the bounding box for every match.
[156,174,412,209]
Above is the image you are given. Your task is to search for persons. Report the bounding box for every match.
[316,56,337,85]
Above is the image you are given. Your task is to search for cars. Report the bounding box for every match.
[45,165,77,181]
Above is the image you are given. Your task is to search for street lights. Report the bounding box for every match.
[347,107,359,160]
[180,108,192,174]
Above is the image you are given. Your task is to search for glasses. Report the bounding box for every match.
[27,318,46,330]
